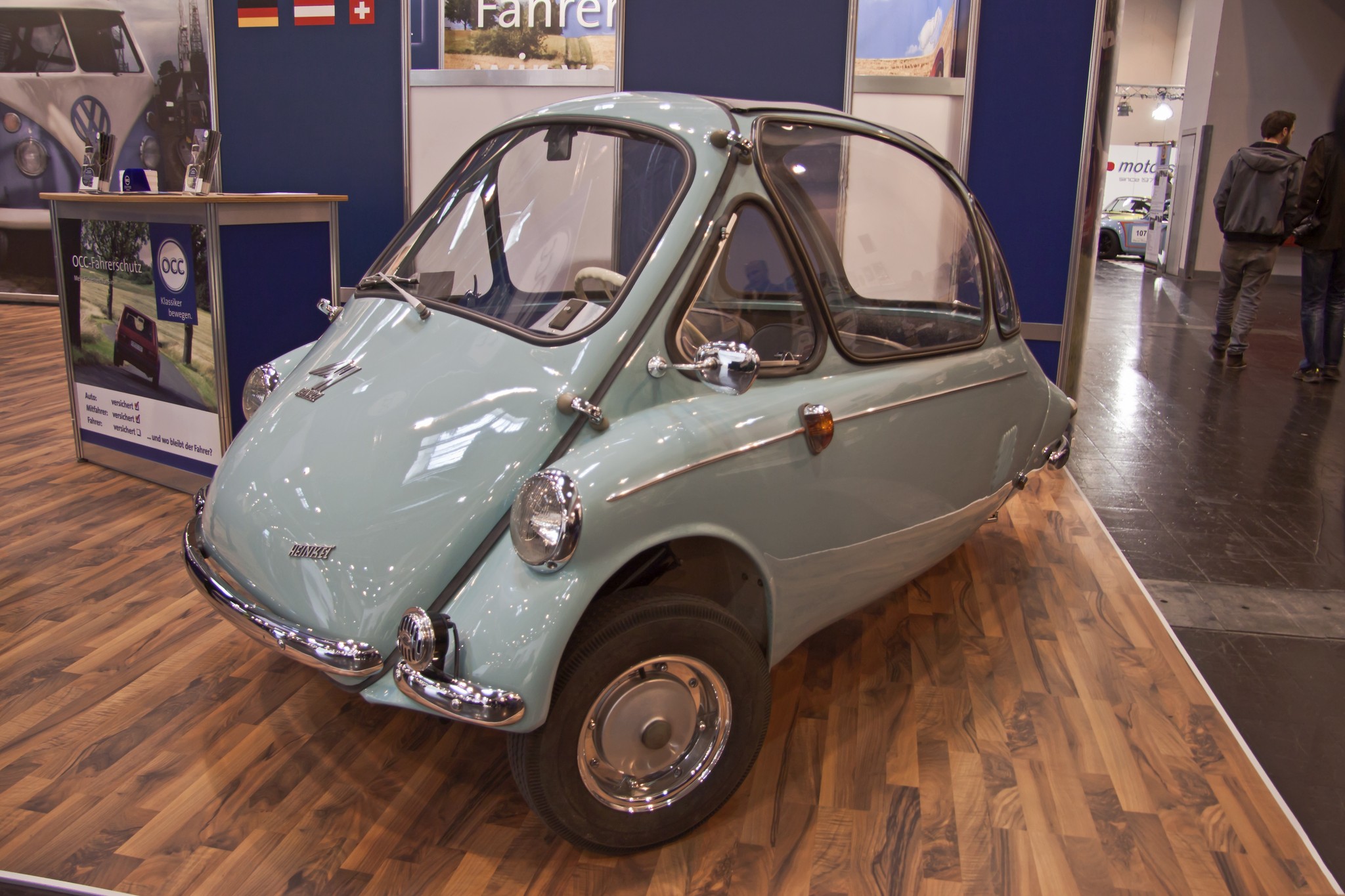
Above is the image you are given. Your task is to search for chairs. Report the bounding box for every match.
[746,323,912,365]
[683,307,751,356]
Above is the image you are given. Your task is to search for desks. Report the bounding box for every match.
[39,192,347,499]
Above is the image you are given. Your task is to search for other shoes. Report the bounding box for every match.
[1227,351,1246,368]
[1208,344,1225,361]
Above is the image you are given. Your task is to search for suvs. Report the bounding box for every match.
[114,303,162,385]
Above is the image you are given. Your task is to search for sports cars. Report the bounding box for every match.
[1098,195,1153,260]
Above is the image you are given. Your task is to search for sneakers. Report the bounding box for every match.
[1321,365,1343,381]
[1292,367,1319,383]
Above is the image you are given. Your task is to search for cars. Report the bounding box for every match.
[180,86,1082,851]
[0,5,165,279]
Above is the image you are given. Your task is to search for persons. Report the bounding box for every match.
[1292,109,1345,384]
[1207,110,1307,371]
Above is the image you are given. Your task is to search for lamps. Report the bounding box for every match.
[1117,95,1133,117]
[1152,87,1174,120]
